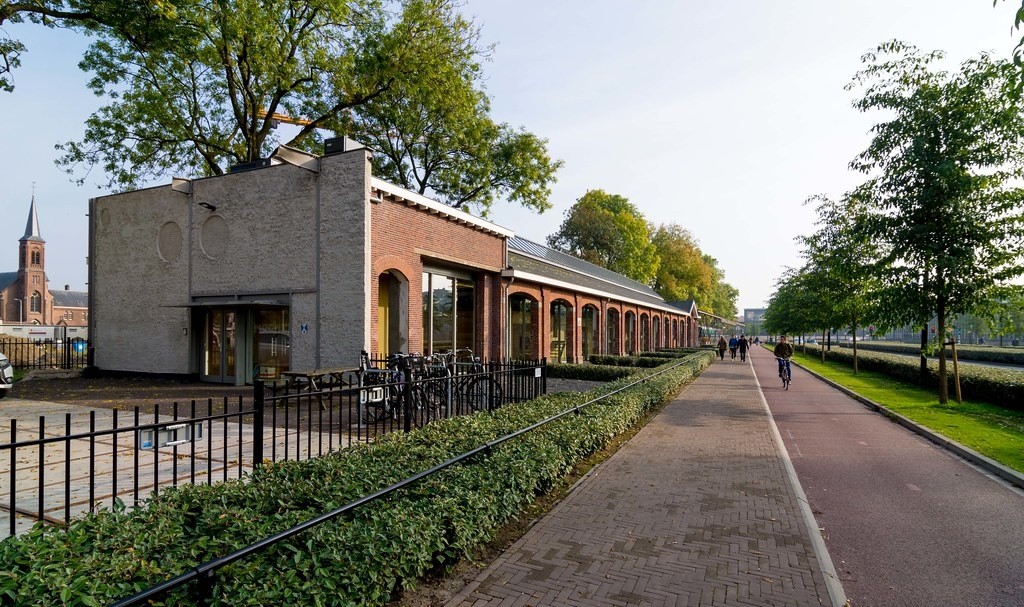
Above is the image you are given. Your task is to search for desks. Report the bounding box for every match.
[279,367,363,409]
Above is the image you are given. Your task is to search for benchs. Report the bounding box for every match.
[264,381,328,406]
[317,384,369,406]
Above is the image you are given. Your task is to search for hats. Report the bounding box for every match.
[740,335,744,337]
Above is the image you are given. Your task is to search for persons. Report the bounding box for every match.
[717,335,727,361]
[738,335,750,363]
[729,334,738,362]
[747,336,760,347]
[774,336,794,385]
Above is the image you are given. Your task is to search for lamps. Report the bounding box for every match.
[198,202,216,211]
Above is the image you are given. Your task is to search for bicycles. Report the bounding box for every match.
[775,357,792,390]
[350,346,505,427]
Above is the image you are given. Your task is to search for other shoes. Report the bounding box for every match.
[732,358,733,361]
[779,373,781,376]
[789,379,791,384]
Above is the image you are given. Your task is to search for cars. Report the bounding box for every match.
[259,330,290,368]
[0,352,13,396]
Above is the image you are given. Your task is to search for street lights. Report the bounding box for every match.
[13,298,23,324]
